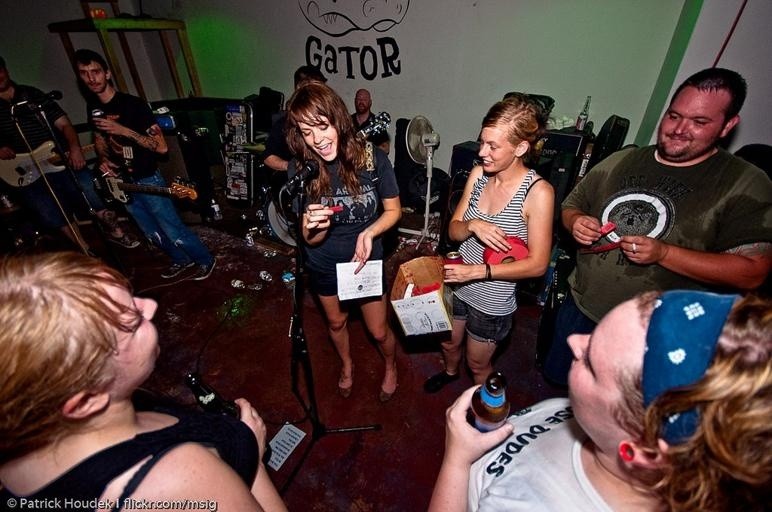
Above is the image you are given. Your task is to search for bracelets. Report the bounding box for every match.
[482,262,495,282]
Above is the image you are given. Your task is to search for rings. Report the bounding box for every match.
[631,242,638,254]
[309,209,315,217]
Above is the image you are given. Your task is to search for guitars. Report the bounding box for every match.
[92,171,197,203]
[1,142,95,186]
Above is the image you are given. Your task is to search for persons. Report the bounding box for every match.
[0,249,287,512]
[543,69,772,391]
[285,82,401,404]
[262,65,328,186]
[71,48,219,284]
[428,291,772,512]
[423,95,555,395]
[350,89,391,156]
[0,59,141,254]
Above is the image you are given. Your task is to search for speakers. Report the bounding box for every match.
[583,114,630,173]
[436,141,483,256]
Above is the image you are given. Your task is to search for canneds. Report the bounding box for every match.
[91,108,105,119]
[245,233,255,247]
[444,250,464,265]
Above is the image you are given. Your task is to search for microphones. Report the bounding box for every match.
[280,158,319,191]
[28,90,63,104]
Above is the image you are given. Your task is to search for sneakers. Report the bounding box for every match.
[424,369,456,394]
[161,260,195,279]
[109,233,140,249]
[194,258,216,281]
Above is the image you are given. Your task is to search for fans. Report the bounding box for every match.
[398,114,445,251]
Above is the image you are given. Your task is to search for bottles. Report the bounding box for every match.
[182,370,271,464]
[468,371,511,430]
[576,94,592,133]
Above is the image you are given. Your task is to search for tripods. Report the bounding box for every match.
[279,190,384,499]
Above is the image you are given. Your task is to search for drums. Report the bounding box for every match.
[267,201,298,246]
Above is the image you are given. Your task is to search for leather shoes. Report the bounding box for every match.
[338,383,353,399]
[379,386,394,402]
